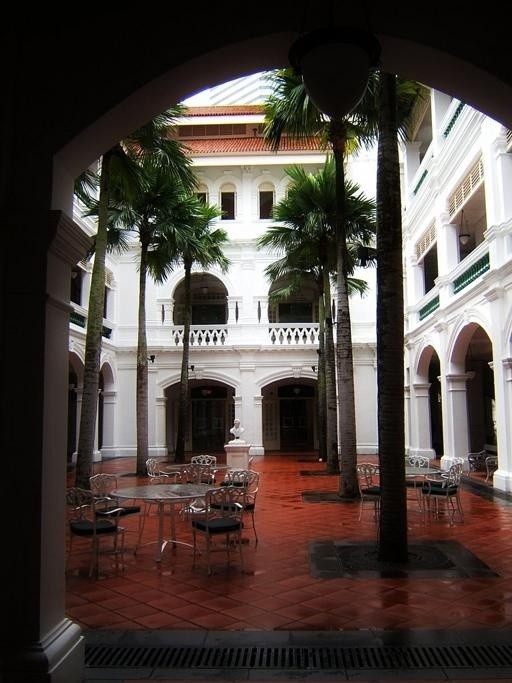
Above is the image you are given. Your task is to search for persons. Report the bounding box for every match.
[230,419,245,437]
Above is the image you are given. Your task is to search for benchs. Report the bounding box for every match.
[466,443,497,482]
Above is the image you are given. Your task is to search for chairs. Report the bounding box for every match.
[66,454,260,575]
[355,455,464,526]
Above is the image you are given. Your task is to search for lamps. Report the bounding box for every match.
[458,208,470,244]
